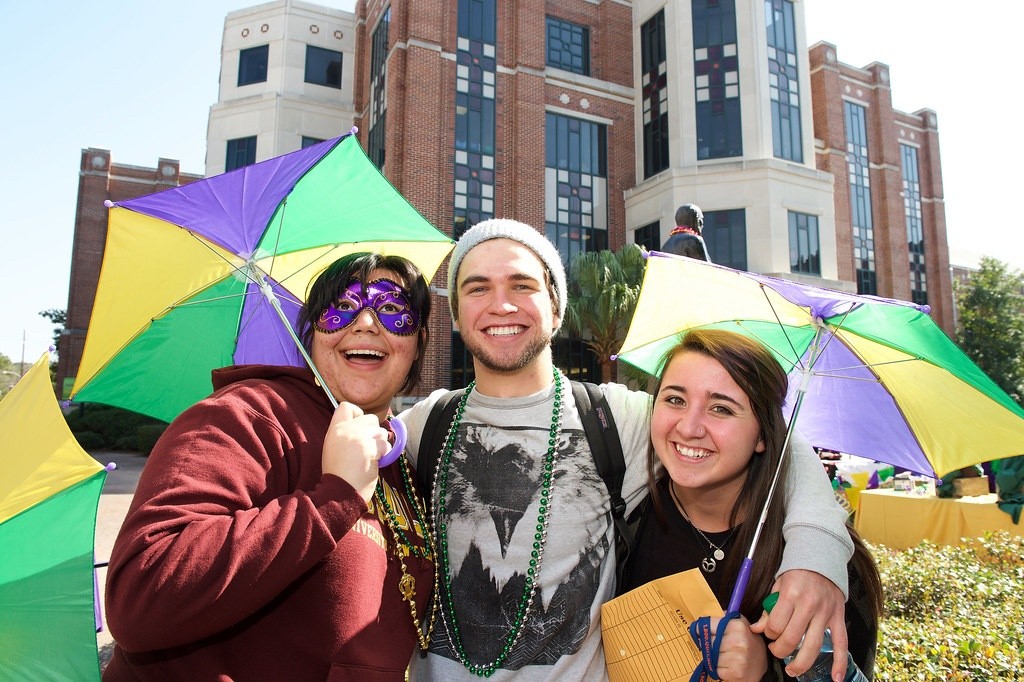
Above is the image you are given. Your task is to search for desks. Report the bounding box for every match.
[822,460,894,523]
[854,489,1024,554]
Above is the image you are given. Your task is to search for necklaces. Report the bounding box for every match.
[431,364,566,678]
[670,226,700,235]
[687,513,748,560]
[373,415,441,651]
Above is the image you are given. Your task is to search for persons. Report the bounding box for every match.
[614,328,884,682]
[391,216,854,682]
[660,203,712,262]
[102,251,441,682]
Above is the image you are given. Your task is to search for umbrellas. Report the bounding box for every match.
[1,344,117,682]
[609,242,1024,619]
[67,135,460,469]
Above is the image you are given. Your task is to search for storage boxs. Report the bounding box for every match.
[953,475,989,497]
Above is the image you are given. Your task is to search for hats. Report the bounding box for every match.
[447,219,567,340]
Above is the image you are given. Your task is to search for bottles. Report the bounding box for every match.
[762,592,868,682]
